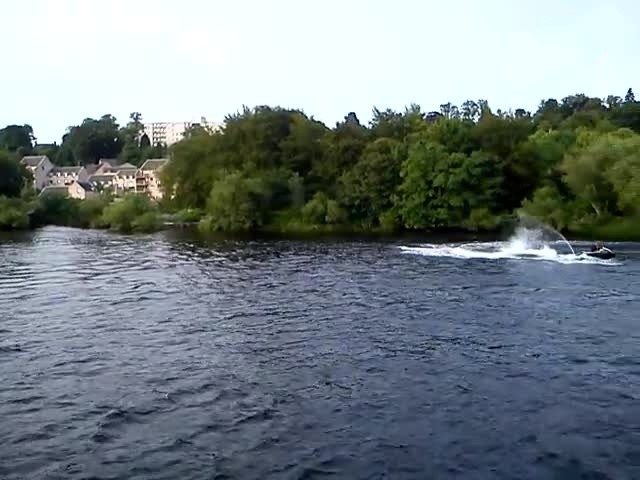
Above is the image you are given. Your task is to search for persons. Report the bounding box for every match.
[591,240,603,252]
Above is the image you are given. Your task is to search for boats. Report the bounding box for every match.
[575,246,616,265]
